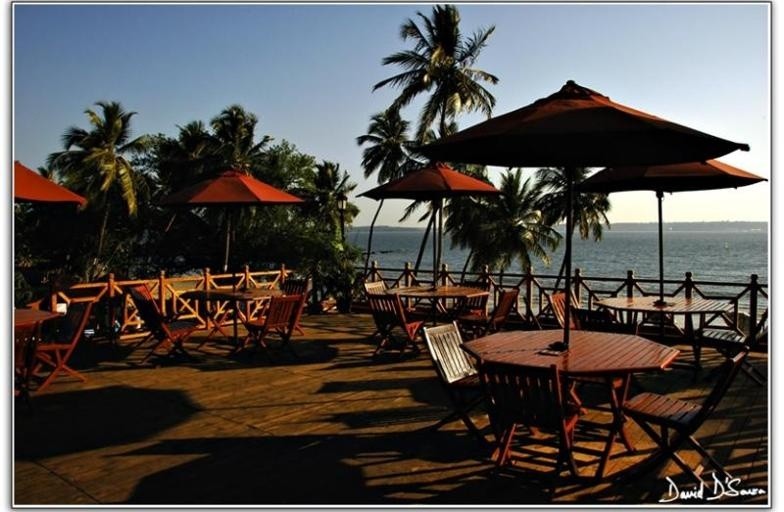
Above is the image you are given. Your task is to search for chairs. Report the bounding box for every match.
[234,294,299,353]
[25,300,93,391]
[274,276,310,336]
[458,290,518,339]
[369,293,425,360]
[571,305,617,333]
[479,358,578,504]
[364,280,416,340]
[445,281,492,333]
[701,308,768,393]
[550,292,578,330]
[423,321,529,466]
[622,345,750,505]
[126,282,195,365]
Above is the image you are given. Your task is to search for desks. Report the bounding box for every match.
[15,309,60,398]
[594,297,735,340]
[179,289,281,356]
[460,330,679,504]
[385,285,490,327]
